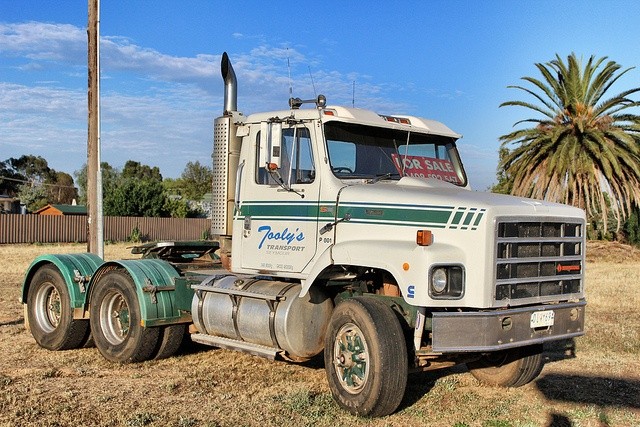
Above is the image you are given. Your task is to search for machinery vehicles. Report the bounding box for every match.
[21,52,586,417]
